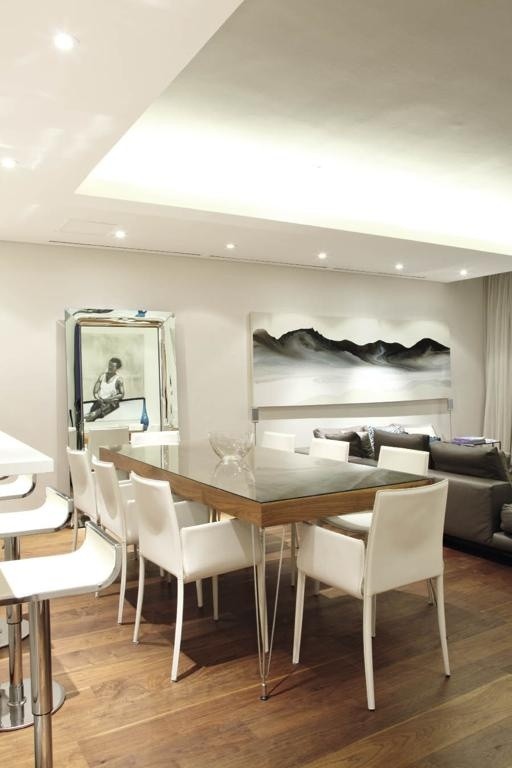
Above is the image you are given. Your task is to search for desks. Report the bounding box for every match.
[96,439,434,700]
[0,431,57,475]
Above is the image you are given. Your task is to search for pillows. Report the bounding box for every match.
[314,425,510,481]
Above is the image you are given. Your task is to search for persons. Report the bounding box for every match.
[85,358,125,422]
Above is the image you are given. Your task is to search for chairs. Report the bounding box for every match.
[255,431,431,597]
[65,447,166,600]
[129,470,264,684]
[291,477,451,714]
[292,445,438,639]
[93,456,211,625]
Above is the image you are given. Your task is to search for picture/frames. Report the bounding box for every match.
[65,307,180,431]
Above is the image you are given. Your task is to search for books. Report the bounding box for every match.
[453,436,485,443]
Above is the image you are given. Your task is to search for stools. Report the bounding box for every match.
[2,520,126,768]
[2,487,75,734]
[2,471,39,649]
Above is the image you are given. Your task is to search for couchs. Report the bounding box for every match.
[297,425,512,571]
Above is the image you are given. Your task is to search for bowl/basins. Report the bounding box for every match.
[208,460,256,489]
[206,429,256,462]
